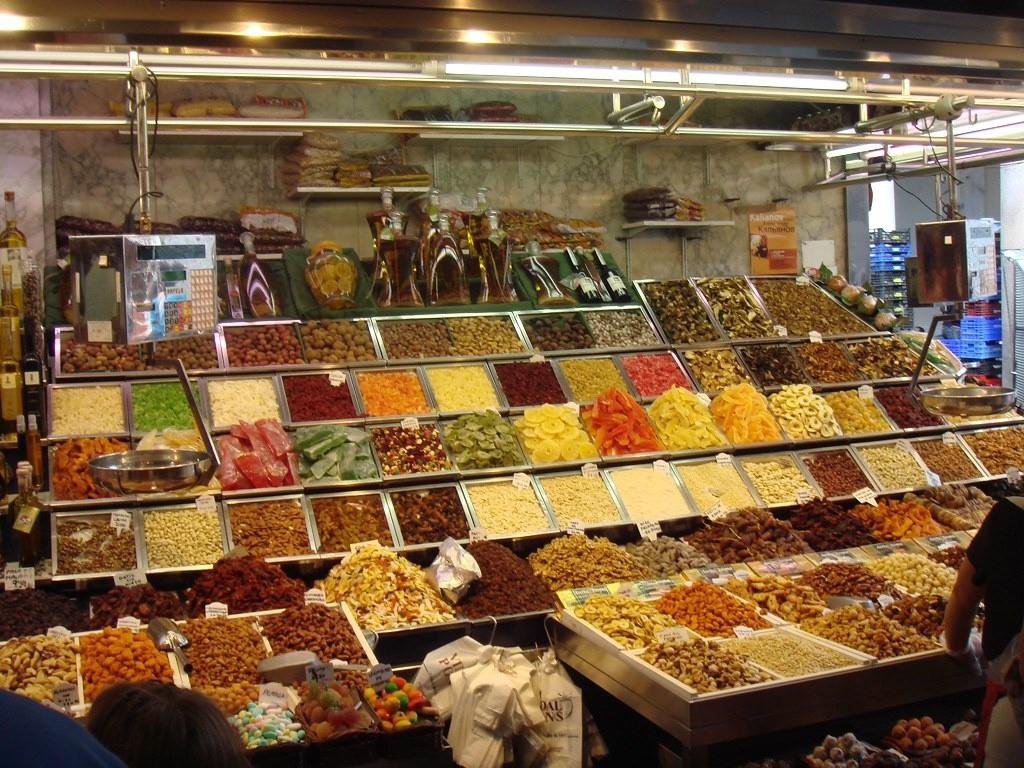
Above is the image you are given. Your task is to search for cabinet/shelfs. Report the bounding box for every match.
[117,129,737,260]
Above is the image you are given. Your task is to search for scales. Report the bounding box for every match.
[913,217,1018,416]
[65,231,230,491]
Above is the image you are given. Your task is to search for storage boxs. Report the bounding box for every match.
[960,318,1001,328]
[885,300,913,313]
[966,374,1001,386]
[960,328,1002,341]
[869,227,911,244]
[996,269,1001,283]
[873,285,907,300]
[961,341,1002,358]
[963,302,1001,318]
[869,241,912,252]
[968,283,1001,300]
[870,261,906,271]
[897,314,914,330]
[961,358,1002,376]
[938,339,960,356]
[870,251,911,261]
[871,271,906,285]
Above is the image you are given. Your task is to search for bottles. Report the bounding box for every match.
[365,186,520,308]
[0,193,44,567]
[240,231,283,321]
[522,240,630,305]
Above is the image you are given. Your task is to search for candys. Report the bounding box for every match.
[227,676,439,749]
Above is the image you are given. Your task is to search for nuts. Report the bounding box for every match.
[52,310,1024,701]
[142,507,226,569]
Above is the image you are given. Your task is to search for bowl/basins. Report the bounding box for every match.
[87,449,213,492]
[920,386,1016,414]
